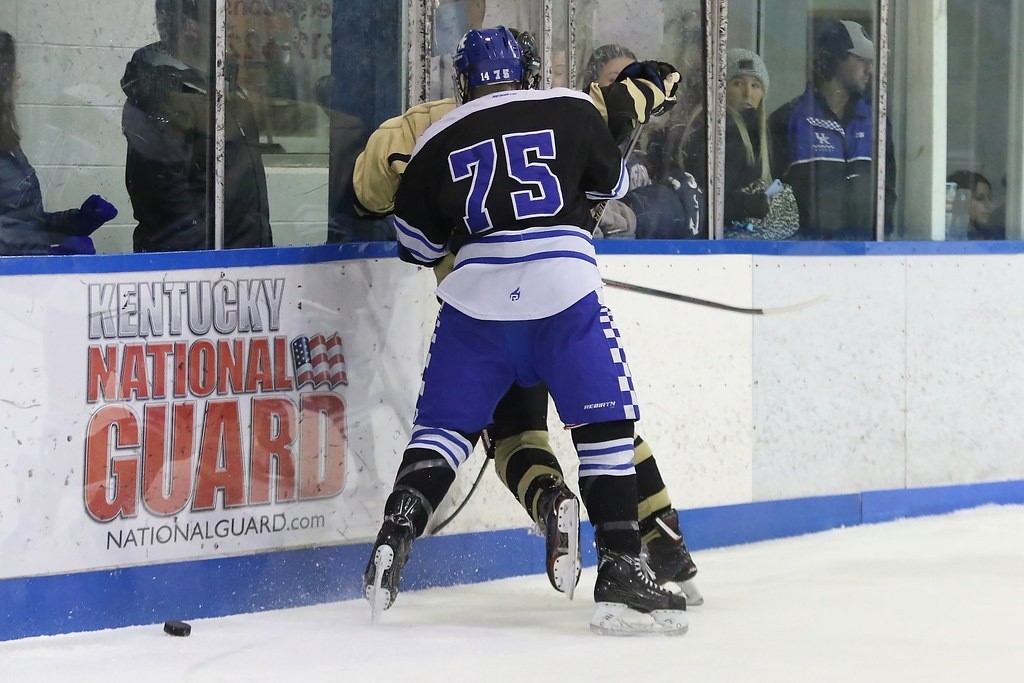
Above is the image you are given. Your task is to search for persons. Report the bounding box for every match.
[358,22,695,635]
[119,0,274,252]
[0,30,119,256]
[949,169,1000,239]
[352,28,705,605]
[575,44,702,239]
[766,18,900,239]
[680,45,802,241]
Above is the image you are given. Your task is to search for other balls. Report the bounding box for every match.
[163,619,193,637]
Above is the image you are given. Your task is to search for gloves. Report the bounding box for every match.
[81,195,118,236]
[592,202,637,238]
[727,189,770,221]
[615,60,681,117]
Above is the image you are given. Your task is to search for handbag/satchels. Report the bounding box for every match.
[724,181,799,240]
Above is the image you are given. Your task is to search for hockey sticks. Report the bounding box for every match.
[430,119,647,538]
[599,273,830,321]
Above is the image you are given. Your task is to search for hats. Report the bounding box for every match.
[818,20,876,60]
[727,48,769,93]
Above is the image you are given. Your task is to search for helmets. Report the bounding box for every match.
[454,26,523,92]
[508,28,543,90]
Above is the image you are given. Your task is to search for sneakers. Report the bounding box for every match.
[361,490,421,625]
[640,509,704,608]
[589,546,690,637]
[536,481,582,600]
